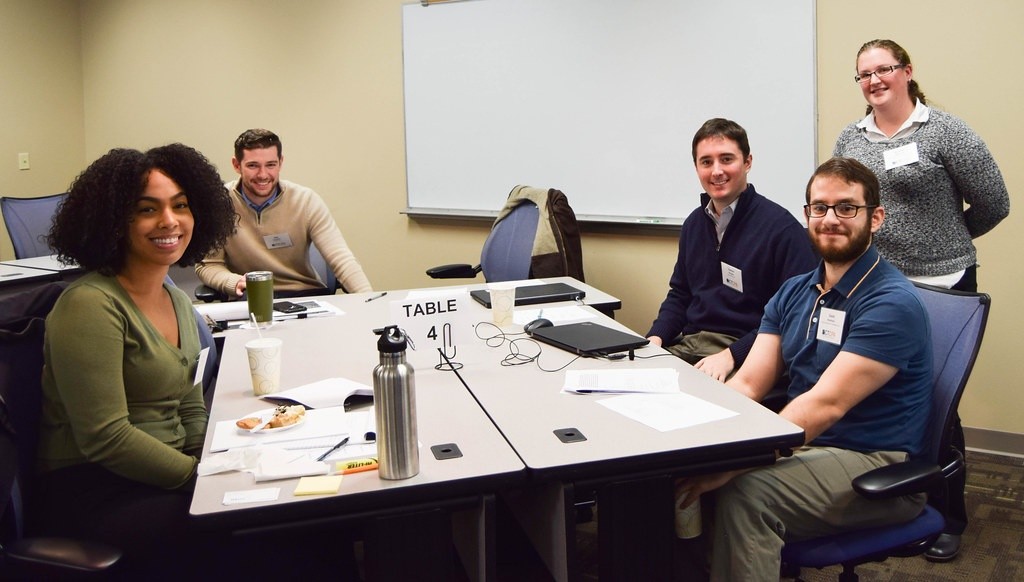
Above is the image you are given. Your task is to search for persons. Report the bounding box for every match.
[38,141,360,581]
[195,129,375,299]
[667,155,940,582]
[833,39,1009,558]
[642,118,816,382]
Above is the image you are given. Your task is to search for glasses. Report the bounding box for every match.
[855,64,902,84]
[804,202,878,219]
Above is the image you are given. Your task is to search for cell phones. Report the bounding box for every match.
[273,302,307,313]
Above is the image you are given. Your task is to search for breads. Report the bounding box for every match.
[235,404,305,429]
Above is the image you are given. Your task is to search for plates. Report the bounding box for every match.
[236,408,304,432]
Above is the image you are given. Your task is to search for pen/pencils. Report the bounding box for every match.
[576,389,646,394]
[317,437,350,460]
[538,309,544,318]
[365,292,387,303]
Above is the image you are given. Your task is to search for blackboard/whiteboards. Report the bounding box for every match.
[399,0,818,231]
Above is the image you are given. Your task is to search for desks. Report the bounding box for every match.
[193,277,806,582]
[0,253,81,288]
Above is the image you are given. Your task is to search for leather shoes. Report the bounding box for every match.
[923,532,962,562]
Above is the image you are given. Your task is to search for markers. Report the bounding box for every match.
[636,218,664,222]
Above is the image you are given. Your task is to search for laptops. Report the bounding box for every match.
[469,283,587,309]
[531,321,651,358]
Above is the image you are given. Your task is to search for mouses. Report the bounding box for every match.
[524,318,553,335]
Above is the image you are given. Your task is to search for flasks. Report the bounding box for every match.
[372,325,420,481]
[244,269,273,325]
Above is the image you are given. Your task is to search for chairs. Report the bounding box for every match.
[0,282,124,582]
[780,281,990,582]
[426,185,575,283]
[0,192,73,261]
[194,241,350,304]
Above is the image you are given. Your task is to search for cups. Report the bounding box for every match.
[488,284,517,328]
[671,483,702,540]
[246,339,282,396]
